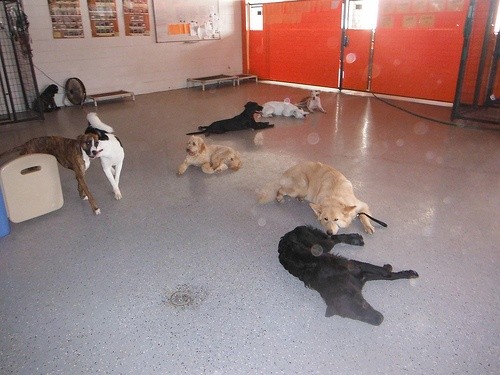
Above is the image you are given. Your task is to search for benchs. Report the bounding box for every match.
[87,90,135,107]
[186,74,258,91]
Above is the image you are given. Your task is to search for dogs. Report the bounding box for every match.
[176,134,242,175]
[293,89,327,113]
[185,101,275,138]
[277,223,418,326]
[85,112,125,200]
[31,84,61,113]
[0,130,101,216]
[255,101,310,119]
[256,159,376,235]
